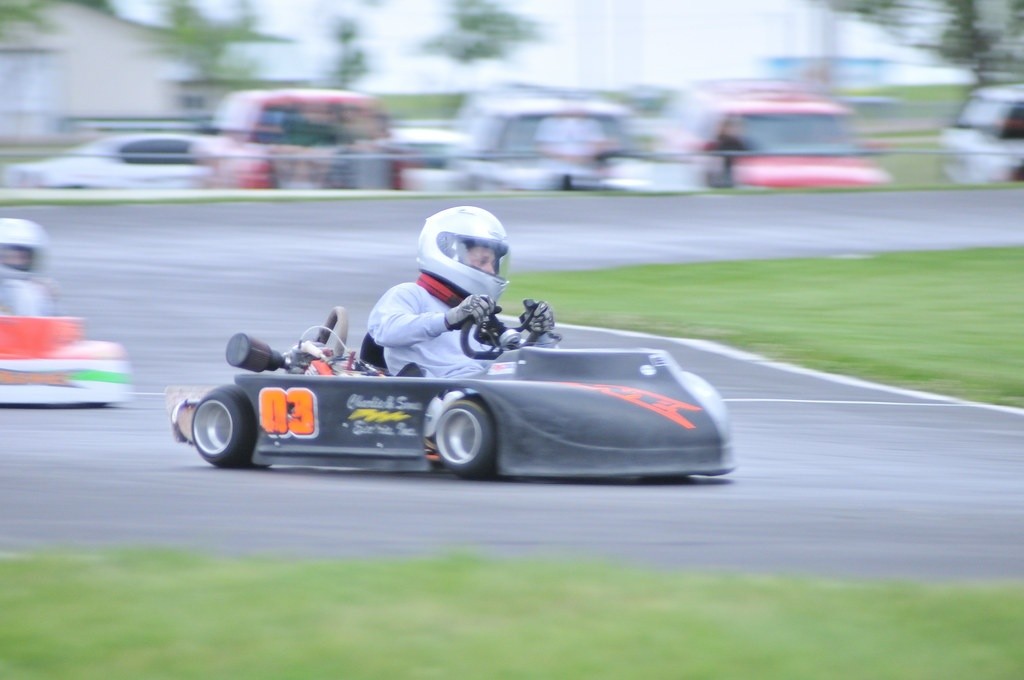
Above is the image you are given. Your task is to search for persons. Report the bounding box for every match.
[697,116,753,189]
[359,206,563,381]
[0,218,56,317]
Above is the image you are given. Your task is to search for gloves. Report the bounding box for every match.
[519,298,554,333]
[445,294,502,331]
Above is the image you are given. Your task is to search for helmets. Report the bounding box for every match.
[416,205,511,302]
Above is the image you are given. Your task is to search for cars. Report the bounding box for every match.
[189,84,398,192]
[448,87,662,195]
[939,82,1023,188]
[668,95,892,187]
[7,130,208,189]
[388,128,479,191]
